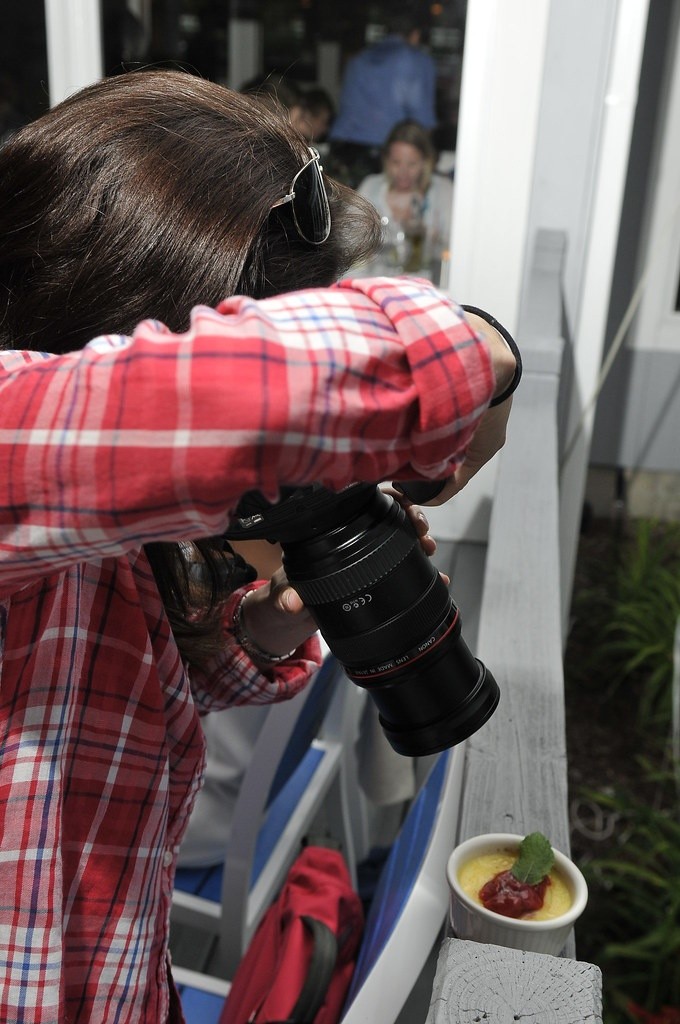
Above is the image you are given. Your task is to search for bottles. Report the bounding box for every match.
[402,197,426,272]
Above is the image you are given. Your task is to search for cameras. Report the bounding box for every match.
[210,477,503,756]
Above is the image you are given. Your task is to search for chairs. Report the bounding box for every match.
[173,741,462,1024]
[170,629,372,986]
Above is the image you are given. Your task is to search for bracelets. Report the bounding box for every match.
[460,305,523,409]
[232,589,296,663]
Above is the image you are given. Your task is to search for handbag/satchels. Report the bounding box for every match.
[218,846,360,1024]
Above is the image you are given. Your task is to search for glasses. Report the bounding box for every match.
[256,148,332,243]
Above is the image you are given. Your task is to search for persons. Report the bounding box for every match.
[325,7,443,188]
[0,71,522,1024]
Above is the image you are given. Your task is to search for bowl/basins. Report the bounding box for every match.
[446,832,589,957]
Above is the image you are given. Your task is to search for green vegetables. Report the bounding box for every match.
[510,831,554,885]
